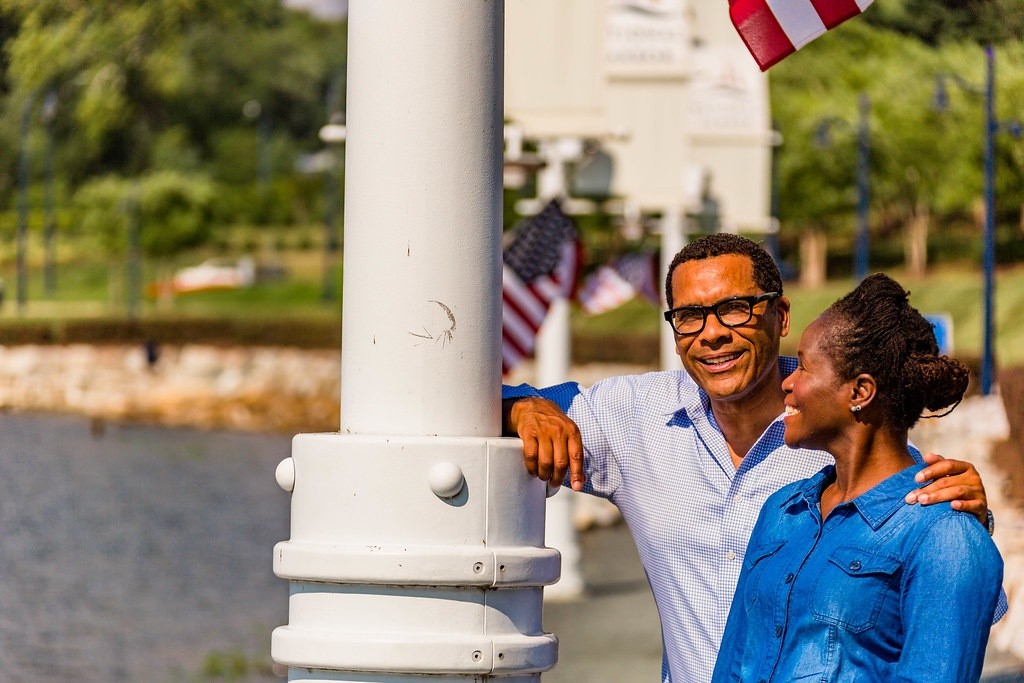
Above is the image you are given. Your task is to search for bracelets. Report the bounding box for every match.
[987,508,994,535]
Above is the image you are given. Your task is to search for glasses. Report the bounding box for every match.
[664,292,780,335]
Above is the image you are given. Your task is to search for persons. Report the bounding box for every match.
[501,232,997,683]
[710,273,1003,683]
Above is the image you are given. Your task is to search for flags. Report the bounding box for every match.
[576,251,659,318]
[499,196,572,376]
